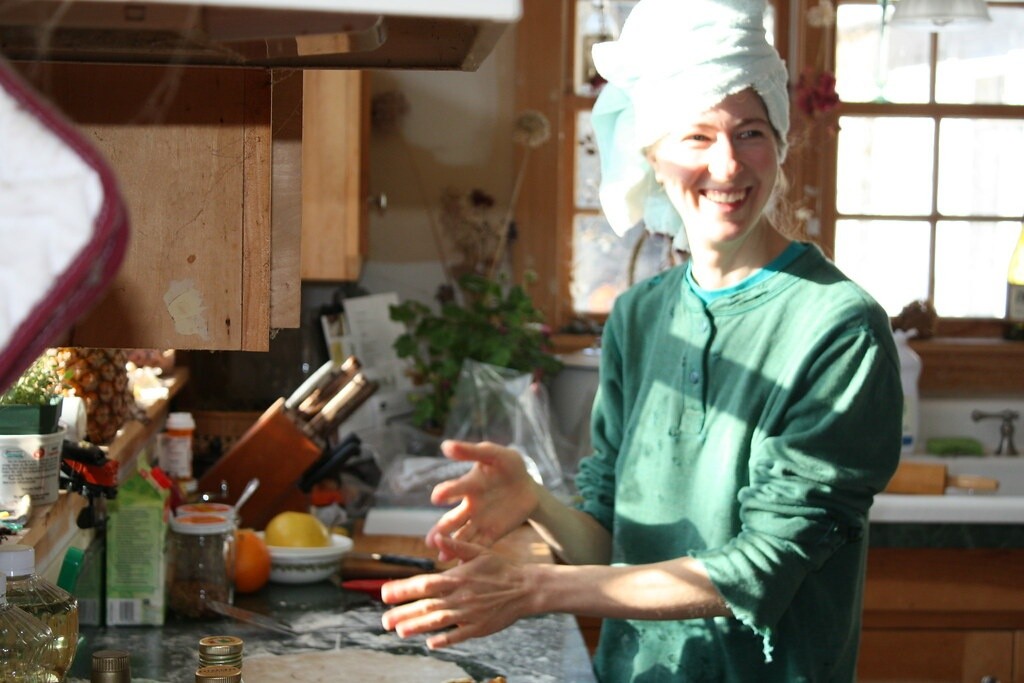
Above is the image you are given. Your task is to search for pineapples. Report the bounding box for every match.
[49,347,130,444]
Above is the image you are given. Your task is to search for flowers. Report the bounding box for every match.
[385,270,566,431]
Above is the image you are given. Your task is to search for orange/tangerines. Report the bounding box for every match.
[226,529,269,592]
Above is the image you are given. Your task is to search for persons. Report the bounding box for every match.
[380,1,903,683]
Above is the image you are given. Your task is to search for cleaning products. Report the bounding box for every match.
[892,328,925,457]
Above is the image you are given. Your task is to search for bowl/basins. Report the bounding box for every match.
[253,530,352,583]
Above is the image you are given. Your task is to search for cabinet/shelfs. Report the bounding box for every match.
[18,59,373,354]
[575,524,1024,683]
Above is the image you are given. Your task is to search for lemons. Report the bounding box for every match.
[266,511,331,548]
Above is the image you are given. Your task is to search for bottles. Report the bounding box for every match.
[193,635,245,683]
[89,648,132,683]
[0,543,81,683]
[166,503,240,619]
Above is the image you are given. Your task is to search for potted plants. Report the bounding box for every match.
[0,346,75,435]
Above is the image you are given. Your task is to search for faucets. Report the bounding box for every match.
[1000,435,1014,457]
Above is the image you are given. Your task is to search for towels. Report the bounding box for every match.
[926,437,983,456]
